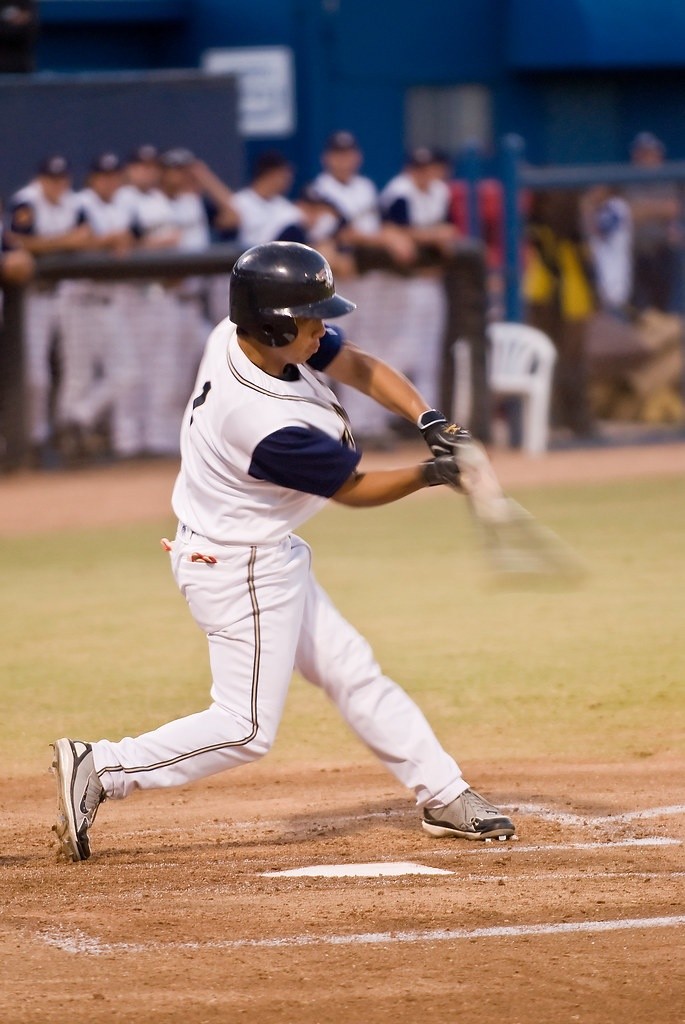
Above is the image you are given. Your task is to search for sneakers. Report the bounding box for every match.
[49,738,114,862]
[422,789,515,841]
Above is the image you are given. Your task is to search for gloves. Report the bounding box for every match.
[162,538,174,552]
[418,409,472,457]
[189,552,218,564]
[419,454,472,496]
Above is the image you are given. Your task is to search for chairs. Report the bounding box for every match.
[451,319,560,460]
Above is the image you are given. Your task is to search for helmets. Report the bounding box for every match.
[229,241,356,346]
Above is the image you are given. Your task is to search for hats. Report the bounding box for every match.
[91,152,124,173]
[38,154,80,176]
[130,144,160,163]
[324,130,357,152]
[408,145,445,166]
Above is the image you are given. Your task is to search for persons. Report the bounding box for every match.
[50,241,516,861]
[0,128,684,456]
